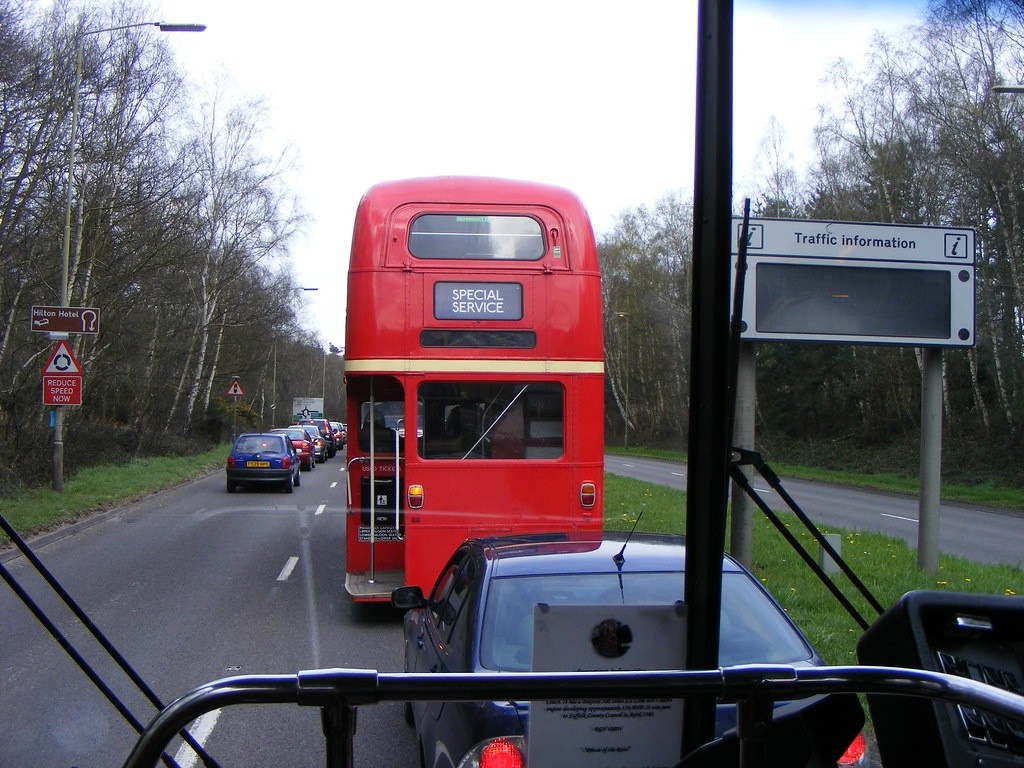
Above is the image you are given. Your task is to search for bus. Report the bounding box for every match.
[336,175,607,607]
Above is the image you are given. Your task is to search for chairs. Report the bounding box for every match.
[359,410,395,452]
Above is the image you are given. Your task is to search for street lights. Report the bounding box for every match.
[322,349,343,418]
[614,311,630,449]
[271,286,319,429]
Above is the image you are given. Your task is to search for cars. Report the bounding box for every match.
[225,432,302,494]
[388,531,873,768]
[287,424,332,464]
[261,428,318,472]
[329,420,347,450]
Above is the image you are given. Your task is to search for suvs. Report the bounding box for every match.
[297,417,338,458]
